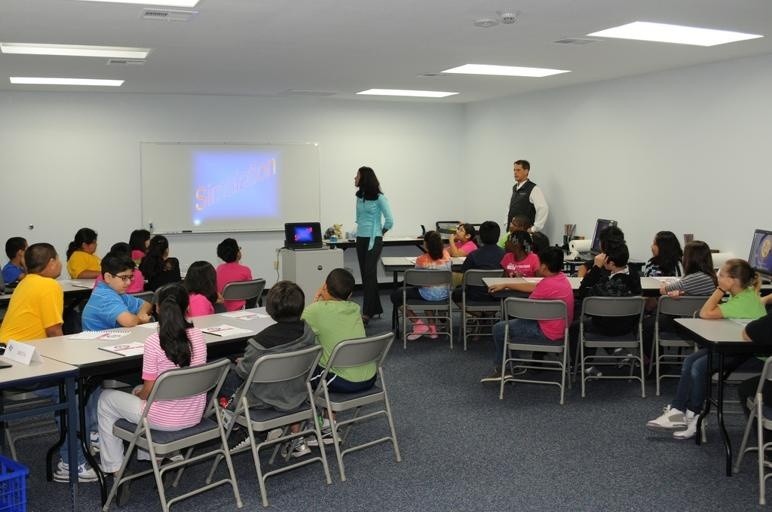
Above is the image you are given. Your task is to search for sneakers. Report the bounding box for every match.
[156,455,184,490]
[671,409,700,440]
[589,367,602,377]
[615,349,630,369]
[226,424,341,458]
[114,471,131,508]
[89,432,101,459]
[52,457,100,484]
[480,361,527,384]
[407,319,429,340]
[424,325,438,338]
[647,404,688,433]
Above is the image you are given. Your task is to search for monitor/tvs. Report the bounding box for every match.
[748,229,772,284]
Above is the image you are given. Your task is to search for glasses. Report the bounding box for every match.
[110,273,134,281]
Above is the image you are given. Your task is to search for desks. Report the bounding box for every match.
[380,256,647,340]
[0,349,82,511]
[27,321,225,511]
[323,232,459,255]
[187,308,277,439]
[0,280,91,336]
[481,275,733,388]
[673,315,772,465]
[71,278,96,308]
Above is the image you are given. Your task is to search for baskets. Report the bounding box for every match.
[0,457,31,512]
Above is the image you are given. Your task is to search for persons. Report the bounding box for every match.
[390,216,772,462]
[1,228,376,507]
[354,166,393,325]
[506,160,549,234]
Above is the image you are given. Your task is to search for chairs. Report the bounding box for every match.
[447,269,504,351]
[286,330,400,483]
[101,357,243,511]
[692,311,766,447]
[574,298,646,399]
[648,296,713,396]
[734,358,771,506]
[396,269,452,349]
[125,278,266,328]
[499,296,571,406]
[207,345,332,506]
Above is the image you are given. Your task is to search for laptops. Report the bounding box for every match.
[569,218,618,266]
[285,223,322,249]
